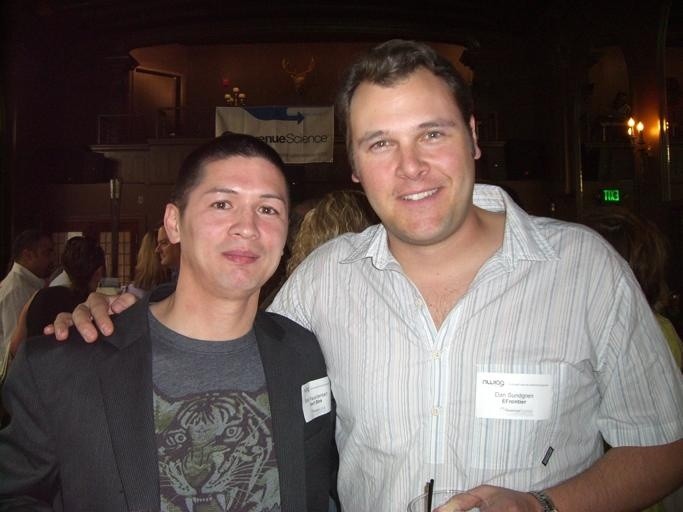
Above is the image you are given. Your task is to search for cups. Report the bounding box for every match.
[407,489,489,512]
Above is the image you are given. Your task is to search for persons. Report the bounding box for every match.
[1,40,683,511]
